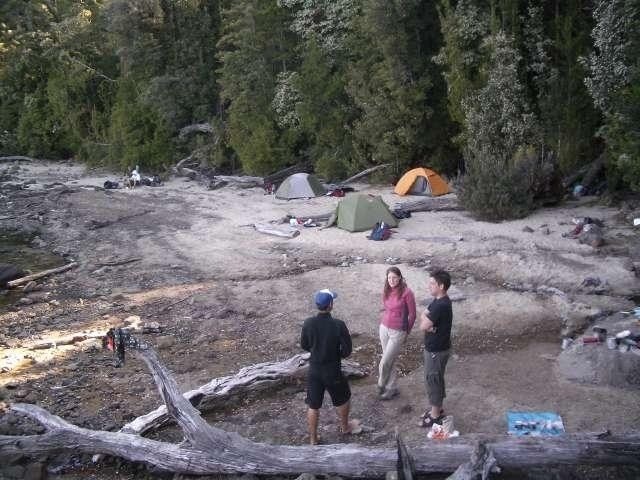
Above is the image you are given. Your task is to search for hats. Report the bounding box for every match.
[316,287,338,308]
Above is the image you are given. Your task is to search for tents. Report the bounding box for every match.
[394,166,454,196]
[275,173,327,201]
[317,191,399,232]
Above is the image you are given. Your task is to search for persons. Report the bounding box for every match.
[374,266,417,400]
[126,165,141,189]
[300,289,360,446]
[416,269,453,427]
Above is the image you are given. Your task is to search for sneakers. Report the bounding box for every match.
[376,386,399,401]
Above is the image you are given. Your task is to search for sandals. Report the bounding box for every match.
[418,408,445,427]
